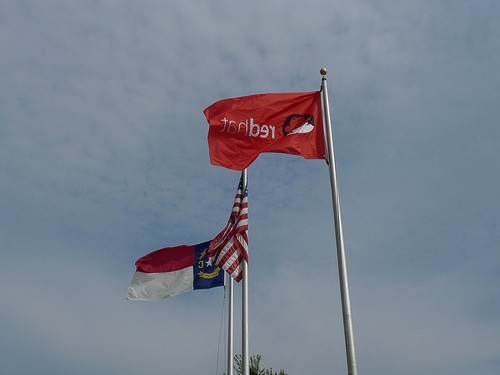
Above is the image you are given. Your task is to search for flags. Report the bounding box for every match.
[203,90,329,171]
[208,172,249,282]
[127,238,225,302]
